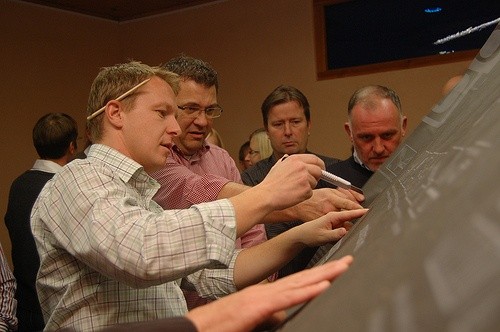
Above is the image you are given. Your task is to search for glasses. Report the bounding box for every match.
[177,103,223,118]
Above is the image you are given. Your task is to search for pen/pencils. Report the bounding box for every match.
[85,77,151,123]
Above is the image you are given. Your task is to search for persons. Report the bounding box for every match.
[241,85,345,280]
[90,254,353,332]
[205,128,274,172]
[30,62,370,332]
[4,113,81,332]
[144,56,364,327]
[287,84,407,275]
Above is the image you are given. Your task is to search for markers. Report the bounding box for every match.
[282,155,366,197]
[309,0,499,83]
